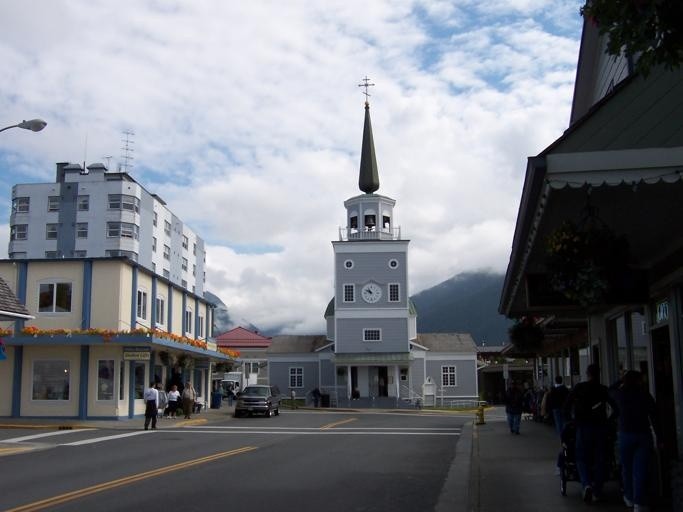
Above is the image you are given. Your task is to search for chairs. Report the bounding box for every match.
[583,486,593,505]
[624,495,633,507]
[634,503,650,512]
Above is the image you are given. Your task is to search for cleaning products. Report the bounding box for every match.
[551,405,620,499]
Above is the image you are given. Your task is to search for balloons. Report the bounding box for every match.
[361,282,382,304]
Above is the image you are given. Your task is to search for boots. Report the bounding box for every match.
[234,384,281,419]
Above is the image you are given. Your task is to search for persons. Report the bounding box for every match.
[169,385,180,419]
[504,378,523,435]
[563,364,620,502]
[606,370,656,510]
[217,380,241,406]
[550,376,569,448]
[144,381,160,431]
[181,381,197,418]
[312,385,320,406]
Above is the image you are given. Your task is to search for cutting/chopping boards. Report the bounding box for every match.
[475,406,486,425]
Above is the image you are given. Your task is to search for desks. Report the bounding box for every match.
[0,119,47,133]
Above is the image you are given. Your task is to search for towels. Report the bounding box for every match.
[321,394,329,407]
[211,392,224,409]
